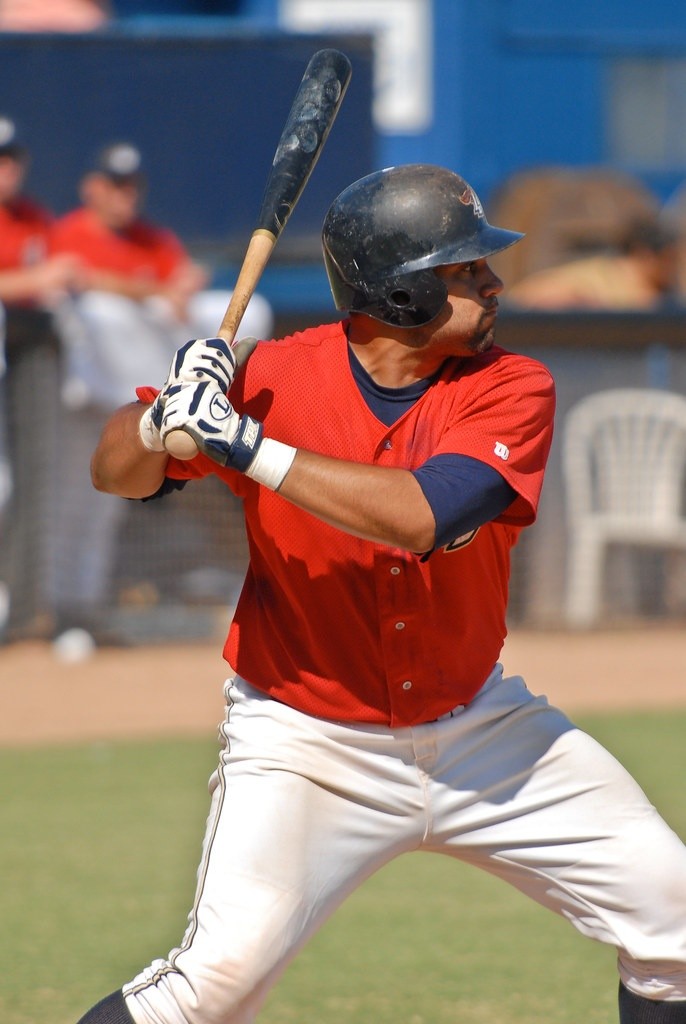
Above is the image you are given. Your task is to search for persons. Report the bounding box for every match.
[0,119,270,656]
[79,166,685,1024]
[509,215,686,312]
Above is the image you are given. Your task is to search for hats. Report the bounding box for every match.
[85,142,143,186]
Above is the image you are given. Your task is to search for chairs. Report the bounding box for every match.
[561,386,686,630]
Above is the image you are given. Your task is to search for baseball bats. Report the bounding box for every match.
[164,48,352,460]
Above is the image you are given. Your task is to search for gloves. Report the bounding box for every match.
[139,336,258,453]
[158,381,298,493]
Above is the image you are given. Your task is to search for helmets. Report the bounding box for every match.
[320,164,526,329]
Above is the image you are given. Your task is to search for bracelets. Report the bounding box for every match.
[245,438,297,491]
[140,407,167,452]
